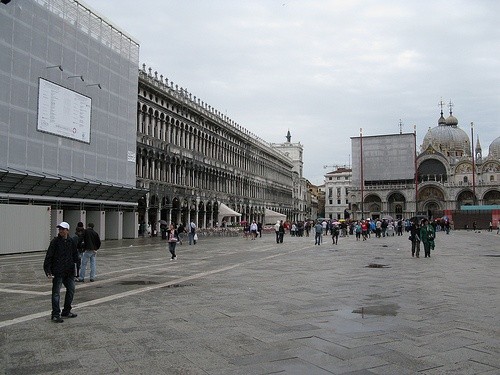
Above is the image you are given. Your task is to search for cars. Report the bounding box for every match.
[405,215,442,232]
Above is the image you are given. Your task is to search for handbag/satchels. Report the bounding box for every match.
[171,237,177,242]
[427,231,433,241]
[193,233,198,240]
[191,227,195,232]
[409,236,412,240]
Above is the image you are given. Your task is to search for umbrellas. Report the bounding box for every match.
[158,220,167,224]
[332,222,341,224]
[339,219,345,221]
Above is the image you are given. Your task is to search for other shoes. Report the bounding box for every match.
[74,277,84,282]
[416,253,419,258]
[412,253,414,257]
[174,255,177,261]
[318,243,320,245]
[90,279,94,282]
[170,258,173,262]
[425,253,427,257]
[428,254,430,257]
[315,242,317,245]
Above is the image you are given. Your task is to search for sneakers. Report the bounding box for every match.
[61,313,77,318]
[51,315,64,323]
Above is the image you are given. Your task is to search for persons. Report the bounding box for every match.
[43,222,78,322]
[284,217,311,238]
[274,220,285,243]
[243,220,262,240]
[168,222,179,261]
[431,218,451,234]
[314,222,323,245]
[356,218,409,241]
[474,219,476,231]
[409,218,435,258]
[312,219,355,235]
[332,225,339,245]
[144,219,196,245]
[74,222,101,282]
[489,220,492,232]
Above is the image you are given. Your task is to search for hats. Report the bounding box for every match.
[56,222,70,231]
[252,220,255,223]
